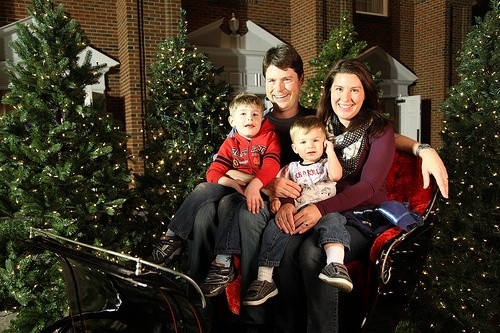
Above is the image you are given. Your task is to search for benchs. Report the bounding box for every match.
[344,149,445,284]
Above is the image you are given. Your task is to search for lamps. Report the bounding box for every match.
[228,13,240,34]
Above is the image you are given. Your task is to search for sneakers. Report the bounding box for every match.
[202,257,238,297]
[244,280,278,305]
[319,262,352,293]
[146,234,186,266]
